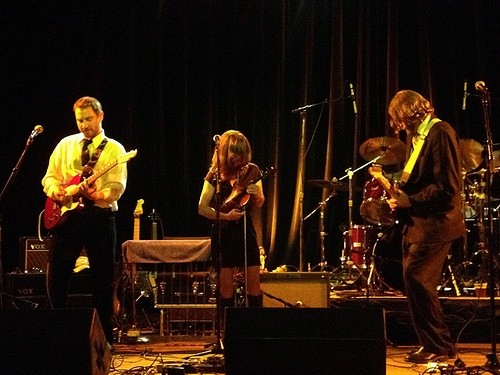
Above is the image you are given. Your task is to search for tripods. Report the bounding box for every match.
[330,95,500,375]
[184,144,222,360]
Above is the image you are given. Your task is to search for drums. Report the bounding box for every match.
[343,225,385,270]
[360,178,399,226]
[373,230,404,292]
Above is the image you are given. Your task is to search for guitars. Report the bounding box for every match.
[44,149,138,230]
[367,164,400,199]
[133,198,144,240]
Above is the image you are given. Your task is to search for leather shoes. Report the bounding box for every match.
[404,347,449,363]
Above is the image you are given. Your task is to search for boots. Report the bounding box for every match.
[214,290,236,353]
[247,290,263,307]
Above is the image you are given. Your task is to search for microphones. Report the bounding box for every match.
[295,301,302,307]
[474,81,489,95]
[24,125,43,146]
[213,134,220,143]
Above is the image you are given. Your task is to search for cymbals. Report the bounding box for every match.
[360,138,407,164]
[458,139,484,172]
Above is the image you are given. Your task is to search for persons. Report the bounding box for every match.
[197,129,265,349]
[387,91,465,365]
[39,96,127,353]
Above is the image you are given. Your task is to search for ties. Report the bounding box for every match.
[81,138,93,166]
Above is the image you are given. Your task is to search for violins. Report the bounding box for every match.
[216,166,275,228]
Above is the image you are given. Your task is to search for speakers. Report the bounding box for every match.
[223,306,386,375]
[0,271,94,307]
[259,271,328,308]
[0,307,112,375]
[18,235,88,271]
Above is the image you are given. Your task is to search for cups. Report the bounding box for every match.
[474,283,487,297]
